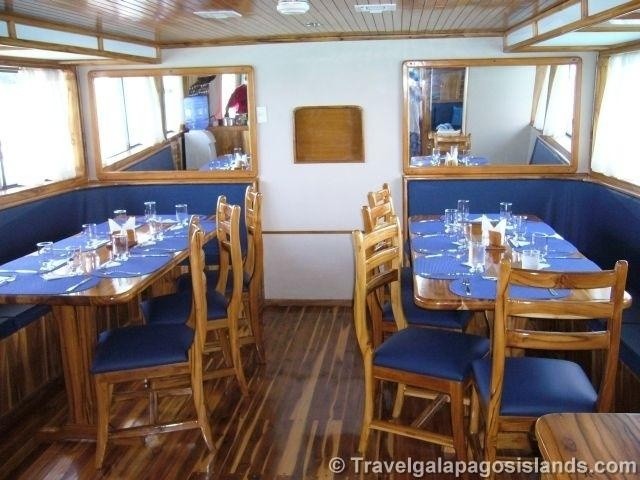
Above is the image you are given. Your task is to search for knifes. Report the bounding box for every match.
[66,277,94,293]
[0,270,37,273]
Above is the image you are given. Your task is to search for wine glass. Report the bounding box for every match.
[66,245,101,276]
[35,241,56,273]
[431,146,479,167]
[207,147,251,171]
[466,243,485,273]
[532,231,548,261]
[442,199,528,241]
[109,202,188,261]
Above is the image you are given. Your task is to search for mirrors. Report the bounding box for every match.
[402,57,582,175]
[87,66,259,180]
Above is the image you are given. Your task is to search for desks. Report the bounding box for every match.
[534,413,640,480]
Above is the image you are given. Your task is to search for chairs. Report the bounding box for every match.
[434,133,472,154]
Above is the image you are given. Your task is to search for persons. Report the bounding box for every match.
[410,74,424,161]
[224,74,249,118]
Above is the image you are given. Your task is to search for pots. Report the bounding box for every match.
[223,112,247,127]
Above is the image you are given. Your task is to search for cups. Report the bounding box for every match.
[82,223,98,246]
[522,250,540,268]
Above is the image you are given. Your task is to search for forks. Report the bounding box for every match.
[462,277,473,297]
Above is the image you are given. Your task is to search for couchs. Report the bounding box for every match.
[115,143,178,171]
[529,134,571,165]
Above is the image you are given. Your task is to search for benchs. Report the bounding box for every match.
[1,180,264,420]
[401,175,640,412]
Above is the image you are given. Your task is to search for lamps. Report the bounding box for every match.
[277,0,310,14]
